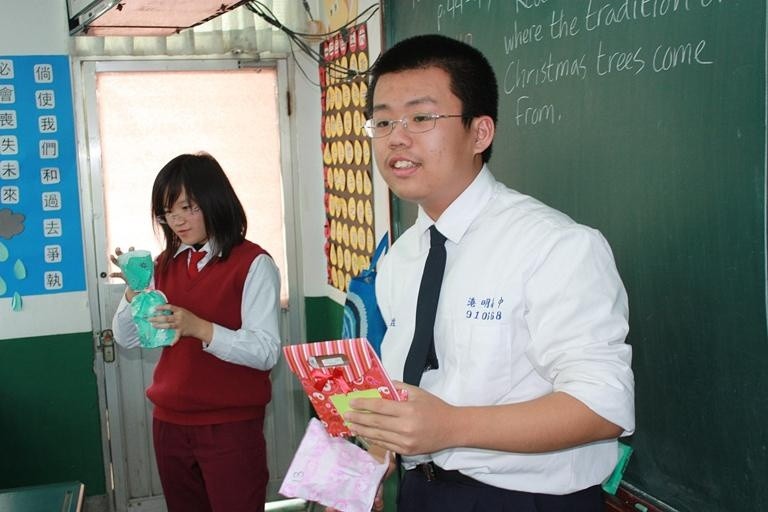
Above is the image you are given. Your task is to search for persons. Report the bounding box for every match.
[111,154,281,510]
[325,32,636,512]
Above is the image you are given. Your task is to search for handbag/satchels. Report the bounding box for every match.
[341,229,388,362]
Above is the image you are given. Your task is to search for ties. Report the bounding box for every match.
[402,225,448,387]
[189,249,208,281]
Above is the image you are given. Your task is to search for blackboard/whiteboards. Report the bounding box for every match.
[381,0,768,512]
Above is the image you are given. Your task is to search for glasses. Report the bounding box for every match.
[361,111,480,139]
[156,208,201,225]
